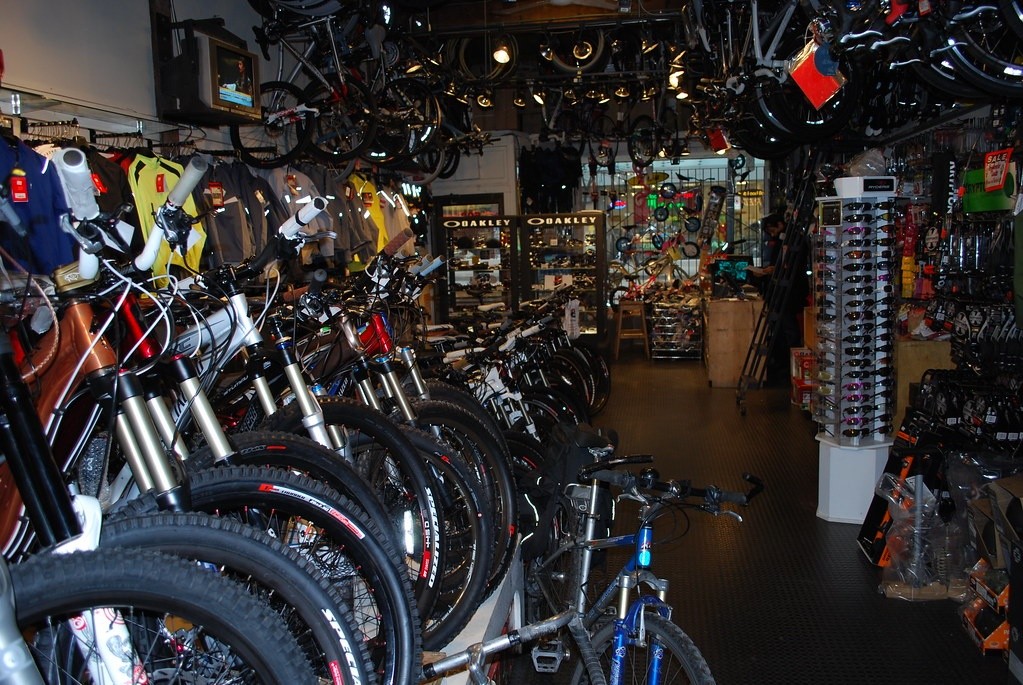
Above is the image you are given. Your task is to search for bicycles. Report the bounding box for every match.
[0,158,1023,685]
[205,1,1023,188]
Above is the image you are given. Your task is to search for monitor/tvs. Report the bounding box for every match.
[174,34,261,118]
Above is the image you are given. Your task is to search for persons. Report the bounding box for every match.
[762,214,811,390]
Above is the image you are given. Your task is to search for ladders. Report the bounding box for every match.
[732,139,830,402]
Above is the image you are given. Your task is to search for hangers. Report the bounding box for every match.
[22,118,339,176]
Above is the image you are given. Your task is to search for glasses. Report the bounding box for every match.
[811,201,896,440]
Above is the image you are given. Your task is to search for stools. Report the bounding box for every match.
[615,299,650,363]
